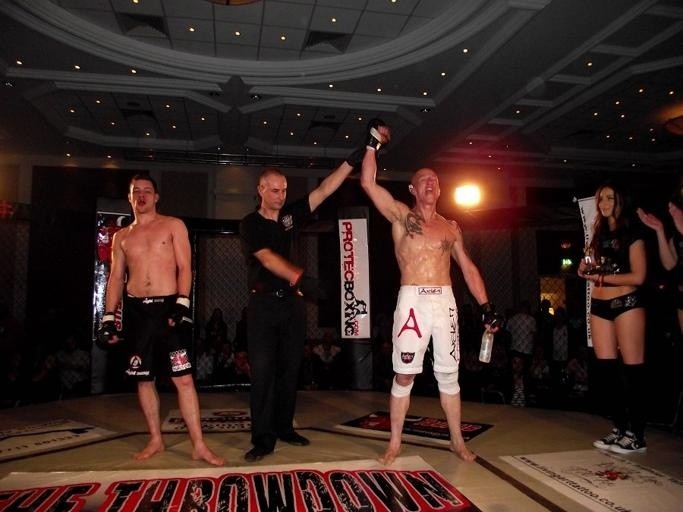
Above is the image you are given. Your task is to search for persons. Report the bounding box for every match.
[0,301,572,406]
[360,124,501,462]
[239,119,389,462]
[577,181,648,454]
[99,173,226,466]
[632,201,683,335]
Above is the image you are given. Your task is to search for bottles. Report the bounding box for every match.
[478,329,495,363]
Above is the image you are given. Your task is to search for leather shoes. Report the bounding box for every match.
[279,432,309,446]
[245,449,273,461]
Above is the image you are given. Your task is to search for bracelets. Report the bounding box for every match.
[595,273,601,287]
[602,274,605,287]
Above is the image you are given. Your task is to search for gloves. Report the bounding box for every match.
[96,312,117,347]
[291,270,328,300]
[167,295,194,330]
[481,302,506,328]
[344,118,390,168]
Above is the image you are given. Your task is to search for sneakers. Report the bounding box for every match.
[610,431,647,454]
[593,428,622,449]
